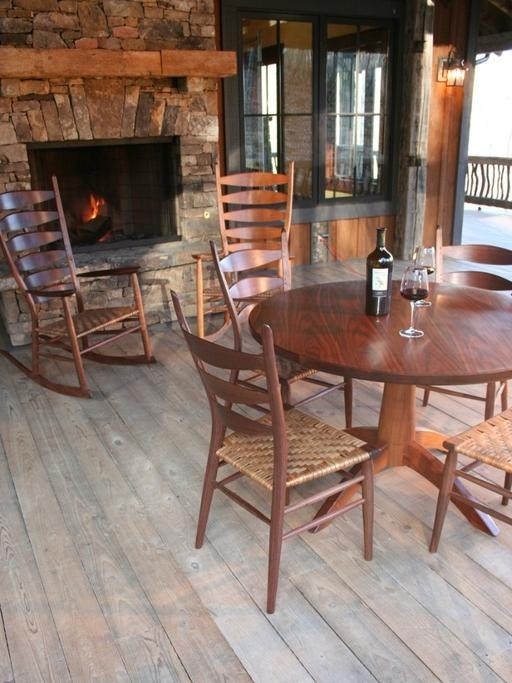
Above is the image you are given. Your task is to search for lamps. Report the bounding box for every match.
[437,42,472,87]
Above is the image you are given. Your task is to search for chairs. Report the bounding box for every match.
[0,176,157,400]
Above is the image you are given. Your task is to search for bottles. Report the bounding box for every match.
[364,227,394,317]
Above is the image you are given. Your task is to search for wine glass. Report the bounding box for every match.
[409,243,437,308]
[398,265,430,339]
